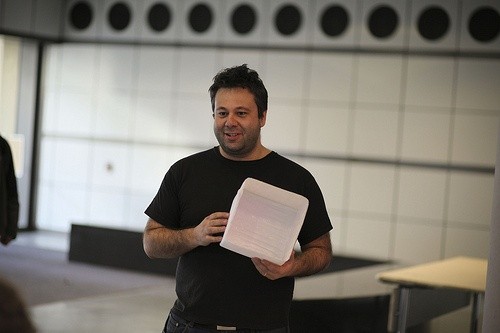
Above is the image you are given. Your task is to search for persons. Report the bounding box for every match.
[143,65,333,333]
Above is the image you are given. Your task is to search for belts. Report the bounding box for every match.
[206,326,253,330]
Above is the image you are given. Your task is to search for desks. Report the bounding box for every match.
[376,257,487,333]
